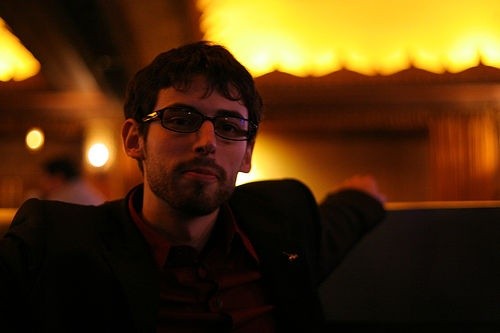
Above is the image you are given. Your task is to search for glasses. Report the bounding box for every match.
[135,104,257,141]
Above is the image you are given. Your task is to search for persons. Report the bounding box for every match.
[1,39,389,332]
[24,148,104,206]
